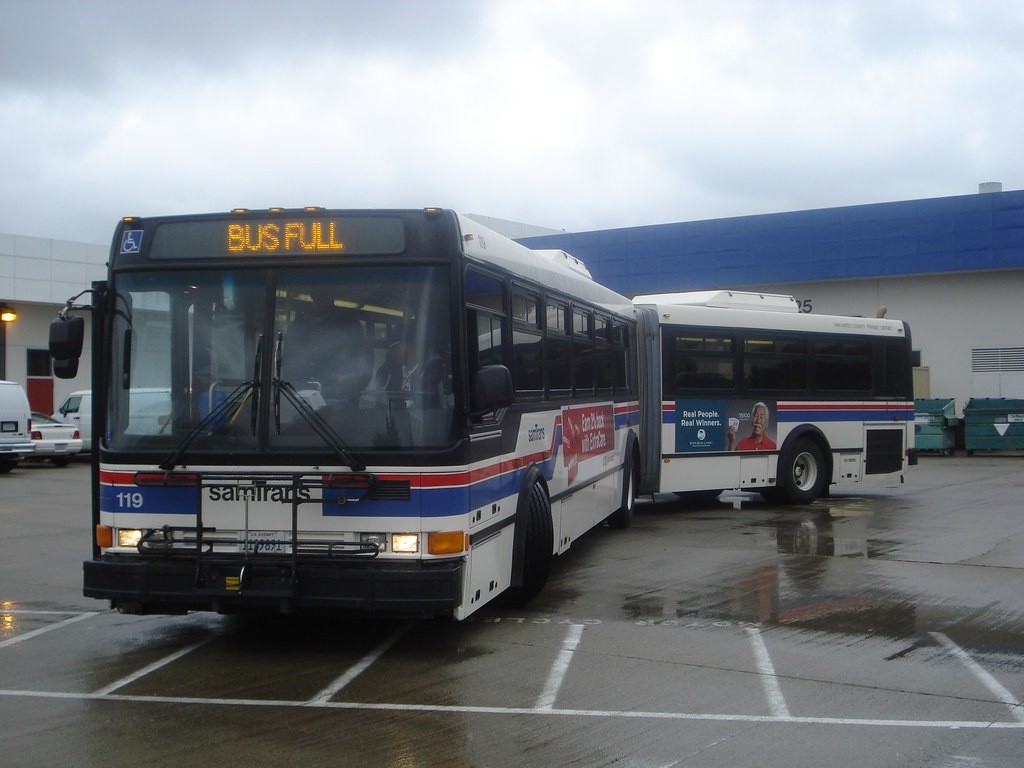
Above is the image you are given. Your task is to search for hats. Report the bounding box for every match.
[382,326,417,347]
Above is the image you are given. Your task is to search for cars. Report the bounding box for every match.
[21,412,84,467]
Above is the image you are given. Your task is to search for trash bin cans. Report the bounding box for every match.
[962,397,1024,457]
[913,397,958,457]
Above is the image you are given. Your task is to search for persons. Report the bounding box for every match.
[728,402,776,451]
[281,279,374,400]
[359,329,444,407]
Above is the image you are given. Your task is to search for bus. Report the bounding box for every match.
[50,204,923,626]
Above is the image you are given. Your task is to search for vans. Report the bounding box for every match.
[51,388,172,464]
[0,381,38,473]
